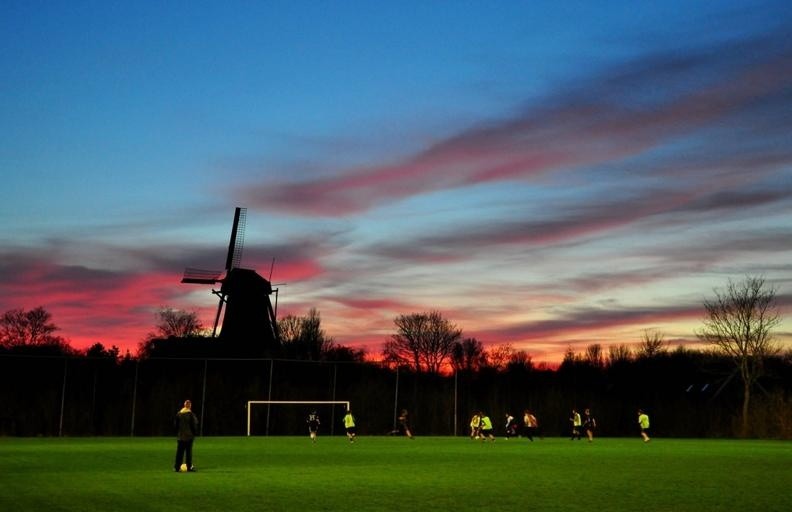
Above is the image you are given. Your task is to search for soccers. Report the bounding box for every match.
[180,464,187,472]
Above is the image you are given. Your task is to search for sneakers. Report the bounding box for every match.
[644,437,651,443]
[310,436,591,443]
[187,466,197,472]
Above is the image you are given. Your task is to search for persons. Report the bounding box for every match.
[636,409,651,442]
[306,409,322,442]
[386,410,415,439]
[342,409,358,442]
[569,408,582,441]
[469,410,543,443]
[582,409,596,442]
[174,399,199,472]
